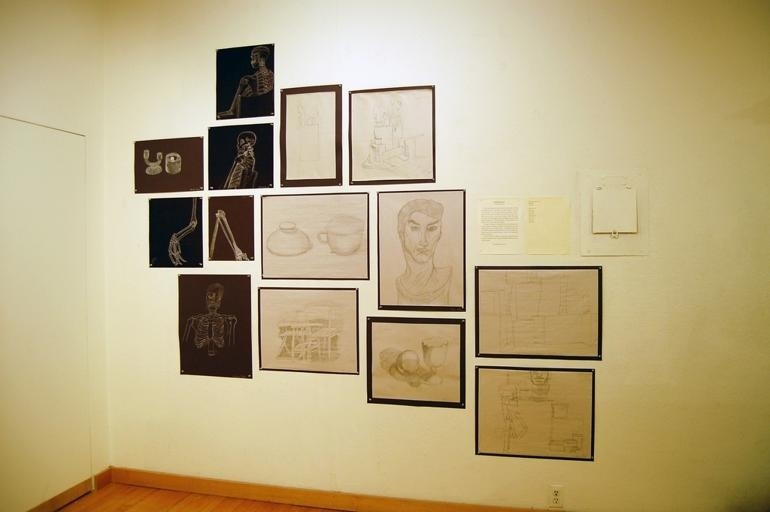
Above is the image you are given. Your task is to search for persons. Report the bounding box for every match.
[217,46,274,118]
[182,283,239,360]
[222,130,257,189]
[391,198,458,304]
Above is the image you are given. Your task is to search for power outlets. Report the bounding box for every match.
[547,484,564,508]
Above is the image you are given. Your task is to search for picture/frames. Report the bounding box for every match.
[204,121,274,190]
[472,263,605,464]
[347,83,436,186]
[375,189,466,311]
[257,286,361,375]
[365,316,467,409]
[277,84,344,187]
[260,192,370,280]
